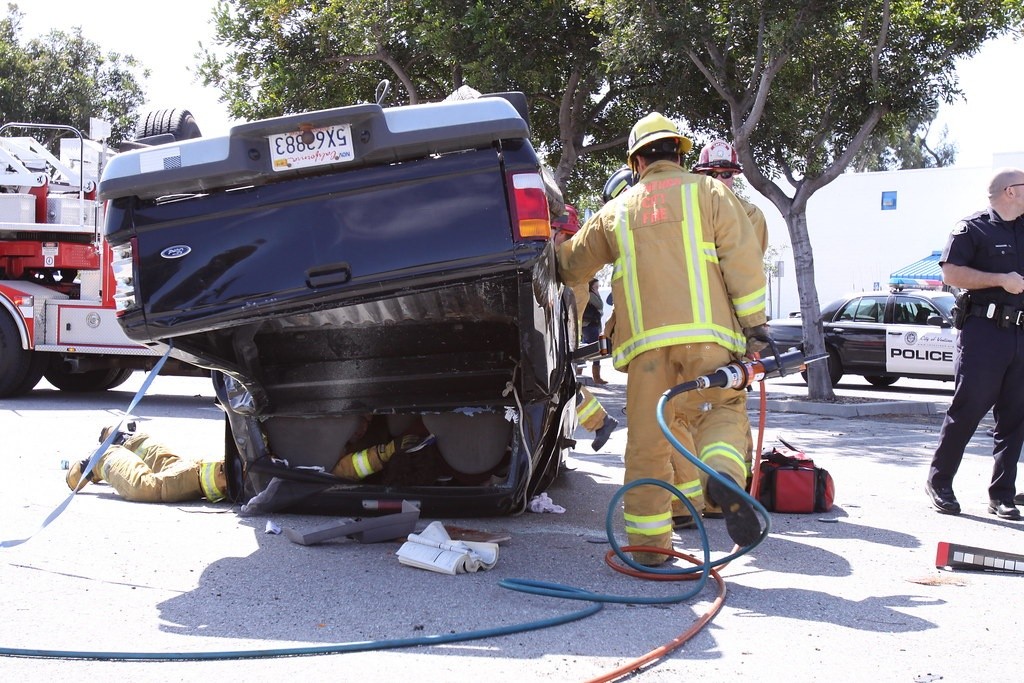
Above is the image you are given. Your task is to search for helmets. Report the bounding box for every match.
[601,165,641,203]
[691,140,742,174]
[552,205,581,235]
[627,111,695,172]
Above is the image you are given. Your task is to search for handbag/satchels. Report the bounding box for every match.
[754,452,835,512]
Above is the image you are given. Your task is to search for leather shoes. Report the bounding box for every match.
[988,498,1020,519]
[925,481,959,514]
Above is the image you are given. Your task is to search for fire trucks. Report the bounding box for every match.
[0,124,164,401]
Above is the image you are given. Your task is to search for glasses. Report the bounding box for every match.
[705,171,734,180]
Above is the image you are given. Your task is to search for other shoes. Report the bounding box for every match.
[708,472,761,546]
[703,512,724,520]
[98,420,137,445]
[66,450,95,493]
[592,414,620,452]
[671,514,700,529]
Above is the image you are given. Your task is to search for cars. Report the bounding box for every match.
[766,277,960,390]
[98,78,603,520]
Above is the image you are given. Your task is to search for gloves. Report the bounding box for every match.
[377,432,422,462]
[742,325,773,353]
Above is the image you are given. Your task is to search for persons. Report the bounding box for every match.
[579,278,611,385]
[550,203,619,452]
[925,169,1024,520]
[553,110,772,567]
[603,292,629,416]
[68,411,421,503]
[600,167,742,531]
[687,139,769,496]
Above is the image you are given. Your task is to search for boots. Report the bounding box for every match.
[591,363,607,383]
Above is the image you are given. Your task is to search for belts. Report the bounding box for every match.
[969,303,1024,326]
